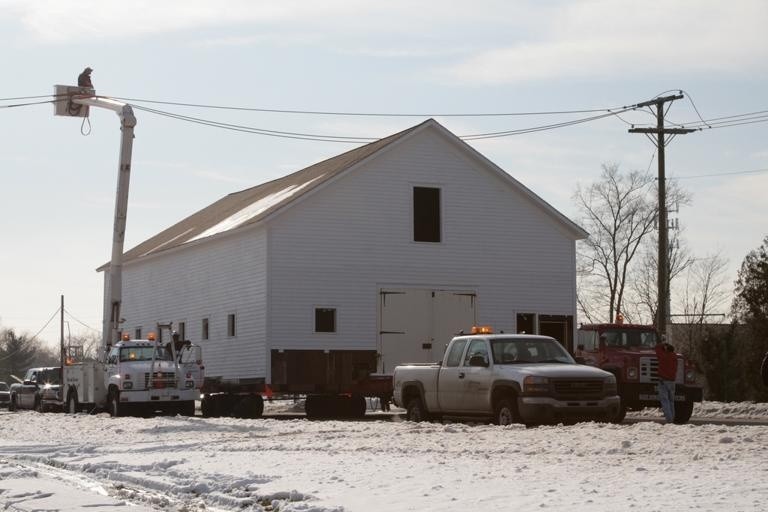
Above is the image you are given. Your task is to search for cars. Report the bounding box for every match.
[0,379,11,409]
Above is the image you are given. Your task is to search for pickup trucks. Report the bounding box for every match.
[10,367,66,414]
[394,330,622,424]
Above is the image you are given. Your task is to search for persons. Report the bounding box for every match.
[166,330,191,352]
[78,66,93,88]
[573,344,586,360]
[655,343,678,424]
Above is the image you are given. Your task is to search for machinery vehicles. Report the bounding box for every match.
[52,84,203,415]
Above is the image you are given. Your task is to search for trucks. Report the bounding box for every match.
[575,315,702,422]
